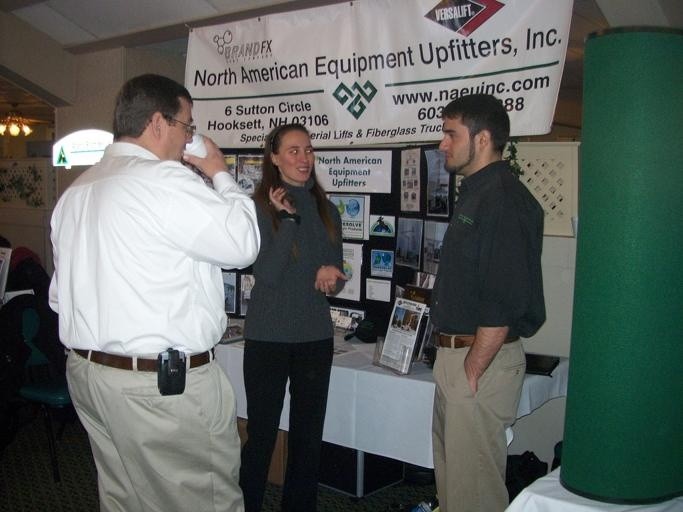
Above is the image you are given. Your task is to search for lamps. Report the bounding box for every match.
[0,103,33,137]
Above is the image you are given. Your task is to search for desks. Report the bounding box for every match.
[214,319,569,498]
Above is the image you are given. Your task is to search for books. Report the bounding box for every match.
[379,296,435,374]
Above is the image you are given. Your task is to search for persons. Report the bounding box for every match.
[428,92,545,512]
[239,124,346,508]
[48,74,263,512]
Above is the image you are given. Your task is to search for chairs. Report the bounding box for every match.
[1,294,72,482]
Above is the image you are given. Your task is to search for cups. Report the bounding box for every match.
[180,134,206,160]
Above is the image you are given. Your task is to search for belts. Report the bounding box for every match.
[74,348,214,372]
[432,333,520,348]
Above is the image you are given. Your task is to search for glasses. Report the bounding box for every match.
[167,116,196,135]
